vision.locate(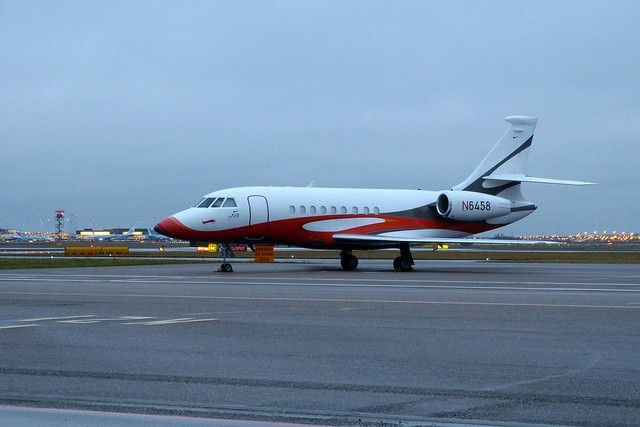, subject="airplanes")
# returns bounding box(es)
[154,115,599,271]
